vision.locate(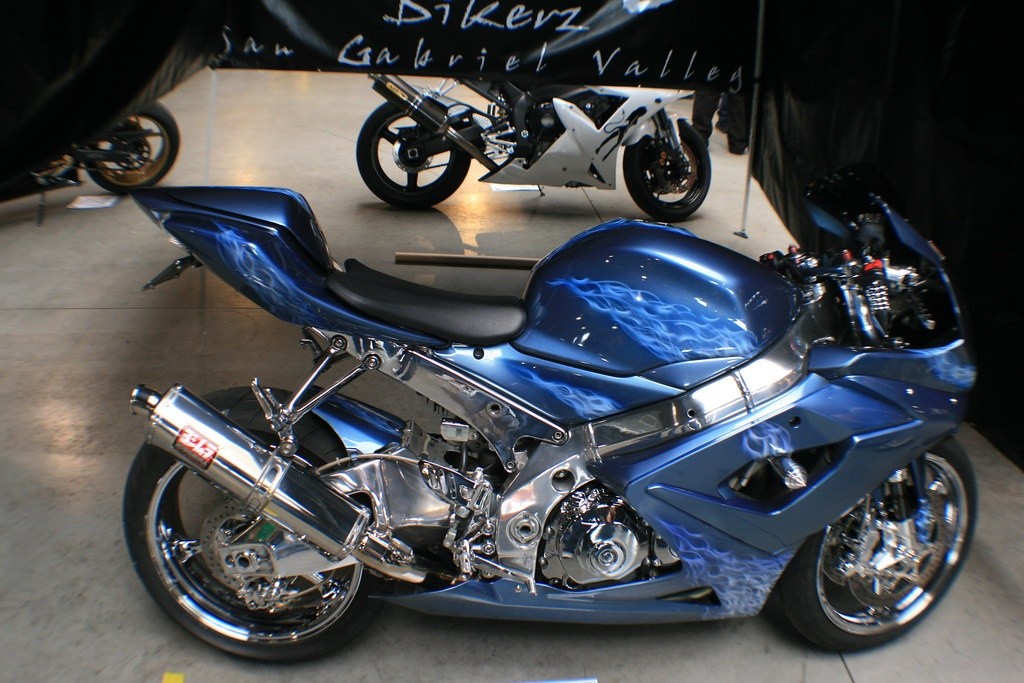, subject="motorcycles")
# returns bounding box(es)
[122,164,992,663]
[356,72,713,221]
[28,102,181,229]
